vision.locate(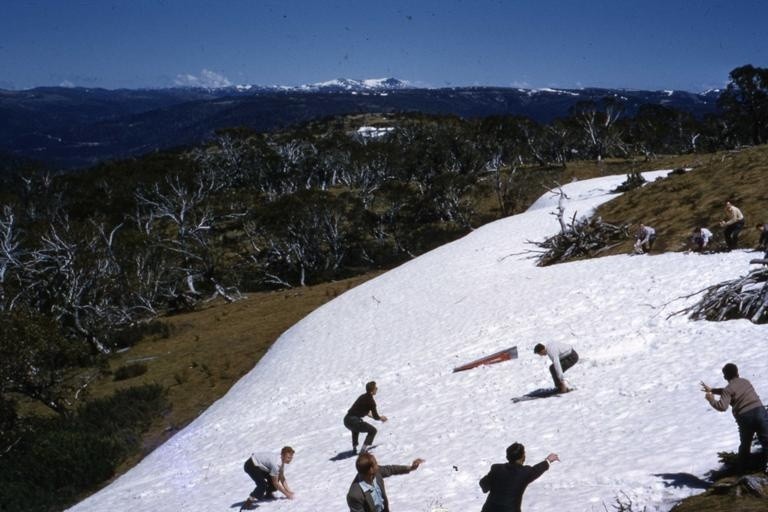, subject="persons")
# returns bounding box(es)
[699,363,767,467]
[717,200,745,250]
[534,341,581,394]
[479,441,561,511]
[343,381,389,456]
[634,224,656,253]
[688,224,715,251]
[241,445,297,510]
[345,452,425,512]
[753,222,768,249]
